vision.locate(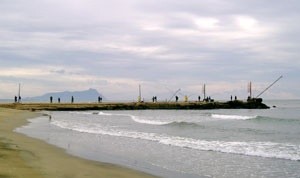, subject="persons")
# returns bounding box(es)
[19,96,21,103]
[71,95,74,104]
[176,96,178,102]
[15,96,17,102]
[50,96,53,103]
[199,96,200,101]
[98,96,100,103]
[58,97,60,103]
